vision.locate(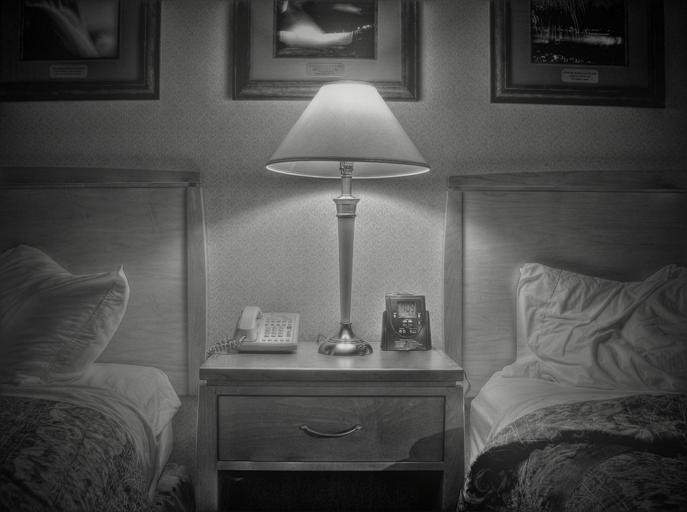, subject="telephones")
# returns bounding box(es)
[235,306,300,351]
